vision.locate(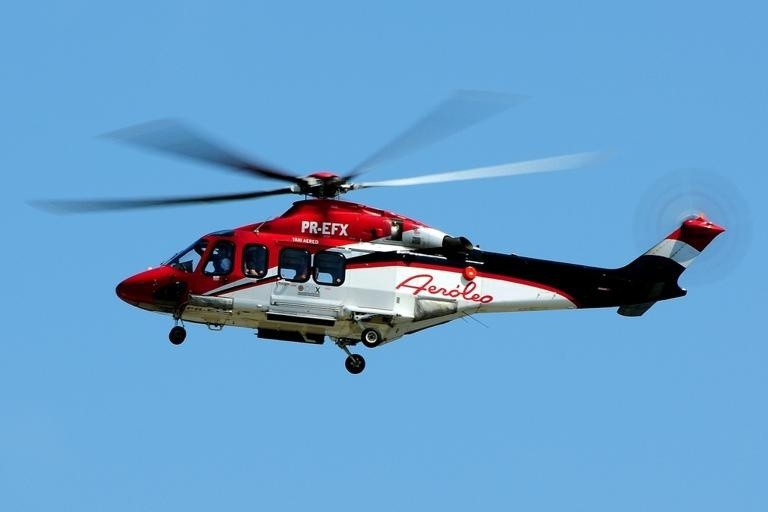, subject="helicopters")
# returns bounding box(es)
[27,71,758,377]
